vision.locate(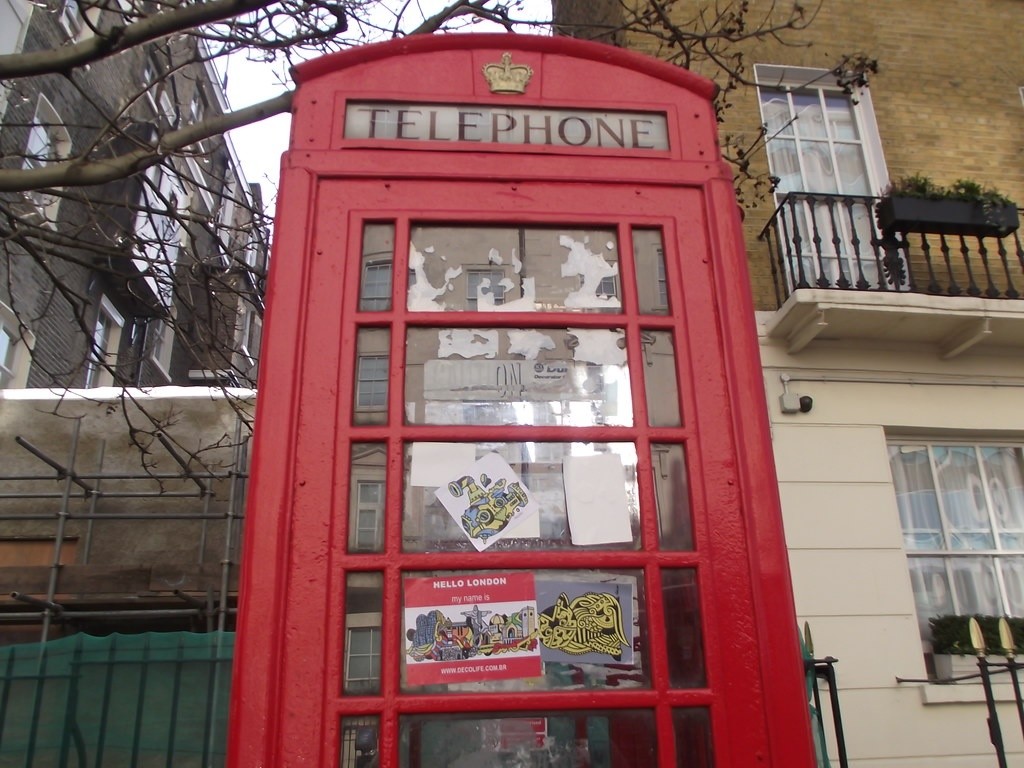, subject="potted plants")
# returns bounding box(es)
[878,171,1020,239]
[930,613,1024,683]
